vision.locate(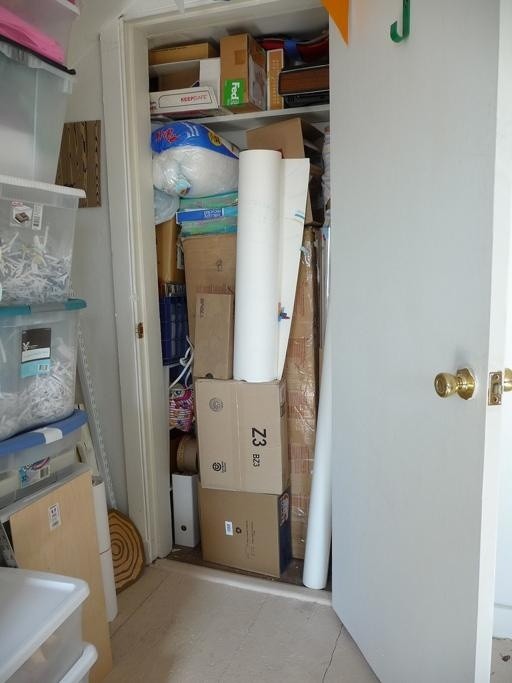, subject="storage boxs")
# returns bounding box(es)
[0,0,101,682]
[147,36,330,583]
